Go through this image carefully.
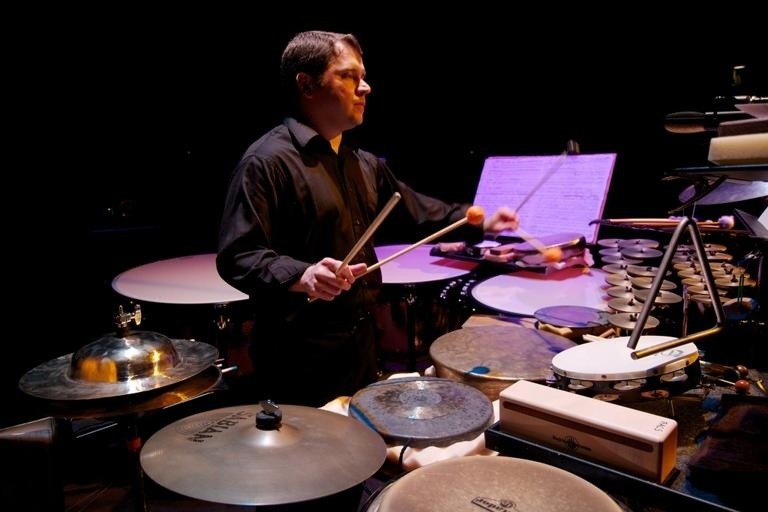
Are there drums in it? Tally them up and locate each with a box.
[369,455,630,512]
[551,334,710,416]
[350,377,495,447]
[429,324,580,403]
[368,244,497,378]
[111,252,256,381]
[470,267,621,342]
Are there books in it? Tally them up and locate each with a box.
[472,150,616,248]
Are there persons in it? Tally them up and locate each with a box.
[216,28,518,399]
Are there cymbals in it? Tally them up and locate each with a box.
[598,237,759,330]
[19,330,224,422]
[140,404,389,507]
